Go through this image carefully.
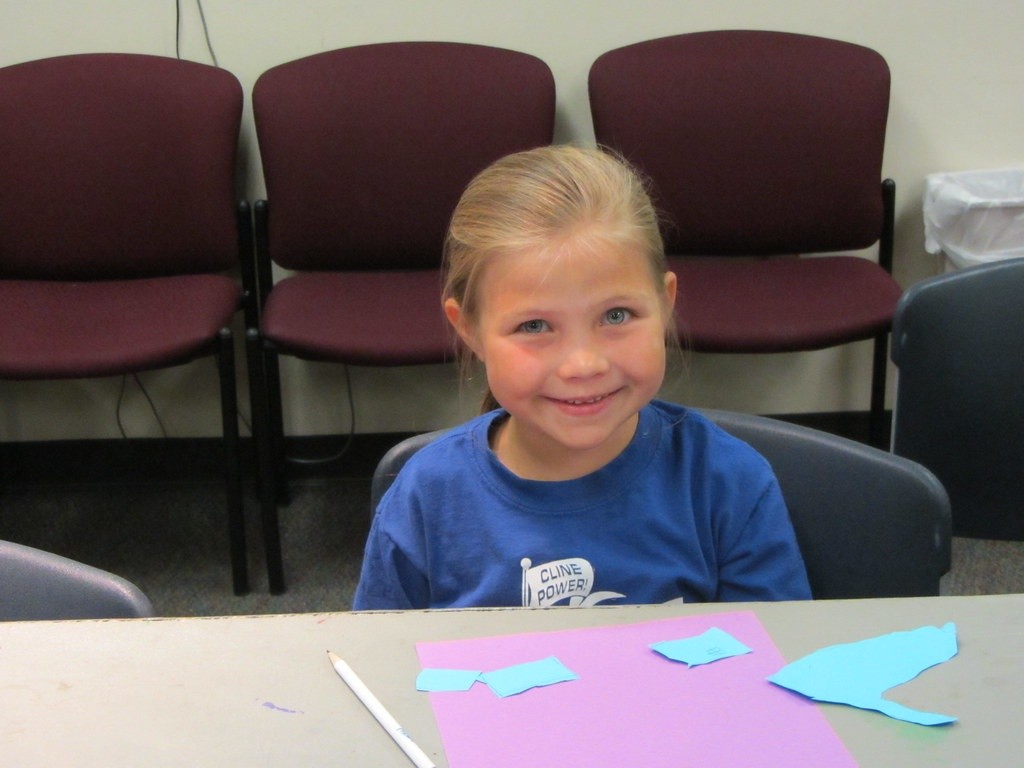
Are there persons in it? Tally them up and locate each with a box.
[353,146,813,613]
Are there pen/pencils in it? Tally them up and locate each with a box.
[326,649,436,768]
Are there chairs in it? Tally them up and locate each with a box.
[585,27,909,448]
[887,257,1024,544]
[366,404,951,600]
[0,52,252,596]
[254,41,557,600]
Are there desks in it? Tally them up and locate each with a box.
[0,594,1024,768]
